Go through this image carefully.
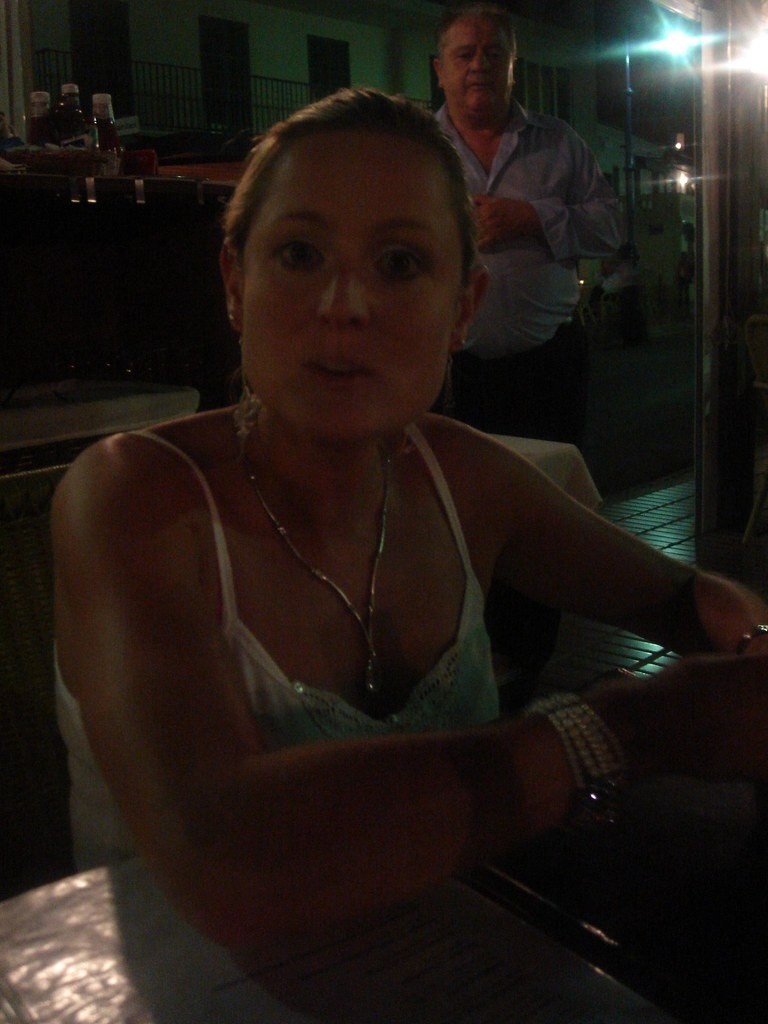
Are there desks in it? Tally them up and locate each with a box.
[485,435,602,710]
[0,772,768,1023]
[1,174,241,411]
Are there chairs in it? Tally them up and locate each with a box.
[577,282,626,329]
[741,312,768,545]
[0,464,78,898]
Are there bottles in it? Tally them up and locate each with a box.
[92,94,121,176]
[52,84,90,148]
[30,91,59,146]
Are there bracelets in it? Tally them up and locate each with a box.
[525,695,628,825]
[737,624,768,655]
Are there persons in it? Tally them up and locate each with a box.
[434,16,620,453]
[47,91,768,951]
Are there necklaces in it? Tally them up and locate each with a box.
[231,405,399,708]
